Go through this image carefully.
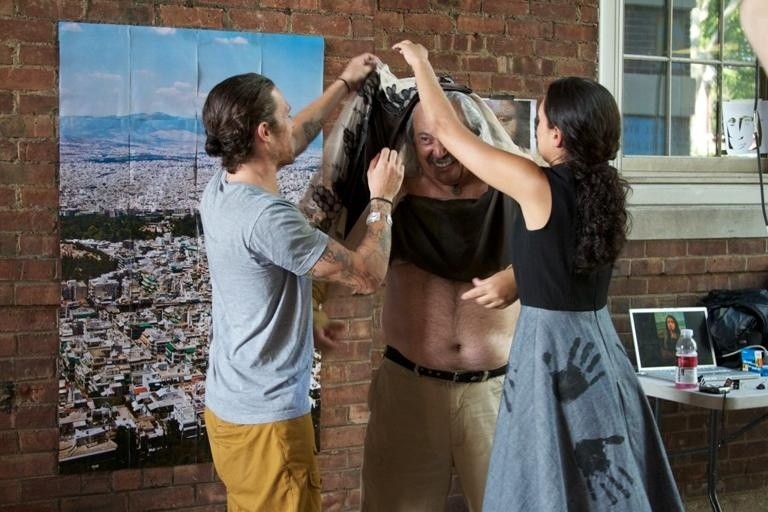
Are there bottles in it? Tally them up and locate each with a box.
[675,327,697,389]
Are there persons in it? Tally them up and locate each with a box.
[391,40,688,511]
[739,0,768,77]
[487,99,517,141]
[665,310,680,353]
[199,51,404,512]
[728,116,753,151]
[315,88,519,511]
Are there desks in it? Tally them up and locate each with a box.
[636,367,767,512]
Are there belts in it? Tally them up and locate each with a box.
[383,345,508,382]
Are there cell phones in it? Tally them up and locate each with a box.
[699,384,731,394]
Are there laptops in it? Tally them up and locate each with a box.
[628,307,761,384]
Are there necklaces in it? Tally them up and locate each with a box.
[448,182,463,196]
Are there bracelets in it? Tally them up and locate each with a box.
[338,75,352,95]
[365,211,393,227]
[367,196,395,208]
